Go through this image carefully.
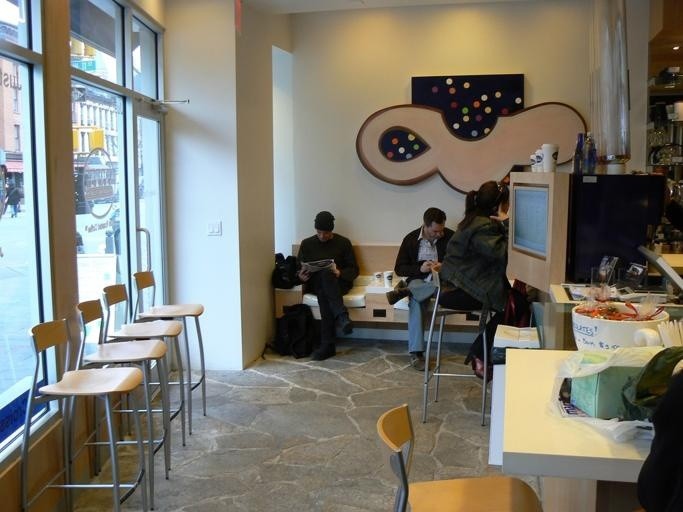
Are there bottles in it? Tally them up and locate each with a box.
[315,211,334,231]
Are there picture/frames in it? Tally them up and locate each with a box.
[485,348,683,512]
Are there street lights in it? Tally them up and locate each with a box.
[272,253,302,289]
[273,304,316,359]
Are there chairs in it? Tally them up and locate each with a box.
[312,347,336,360]
[342,319,352,335]
[409,354,431,371]
[386,288,409,305]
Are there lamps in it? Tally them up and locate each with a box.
[65,298,168,509]
[95,283,186,470]
[376,403,543,512]
[126,272,207,439]
[19,317,149,510]
[415,264,491,427]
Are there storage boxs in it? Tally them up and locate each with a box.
[273,243,515,329]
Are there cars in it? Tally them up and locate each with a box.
[528,143,559,173]
[588,264,641,301]
[650,103,682,122]
[383,270,393,289]
[374,271,382,286]
[570,302,670,351]
[668,67,680,73]
[647,217,682,253]
[646,128,671,166]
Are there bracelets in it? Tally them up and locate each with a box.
[672,156,682,163]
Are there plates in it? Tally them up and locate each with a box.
[543,283,683,348]
[646,83,683,168]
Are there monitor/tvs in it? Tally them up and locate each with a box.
[301,259,334,273]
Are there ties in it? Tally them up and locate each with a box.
[506,171,570,294]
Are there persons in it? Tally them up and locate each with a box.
[386,207,456,371]
[297,211,359,361]
[7,182,20,217]
[438,180,510,382]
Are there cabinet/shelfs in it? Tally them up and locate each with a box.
[574,132,596,176]
[665,178,682,205]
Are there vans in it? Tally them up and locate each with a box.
[464,353,490,379]
